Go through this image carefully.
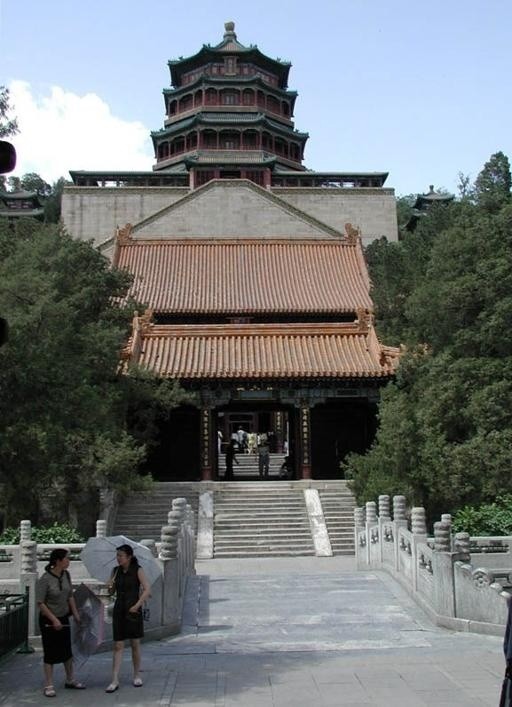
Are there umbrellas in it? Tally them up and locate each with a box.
[79,535,163,591]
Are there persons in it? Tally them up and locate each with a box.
[218,425,288,479]
[36,545,151,697]
[499,596,512,707]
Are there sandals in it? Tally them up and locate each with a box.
[44,677,144,696]
